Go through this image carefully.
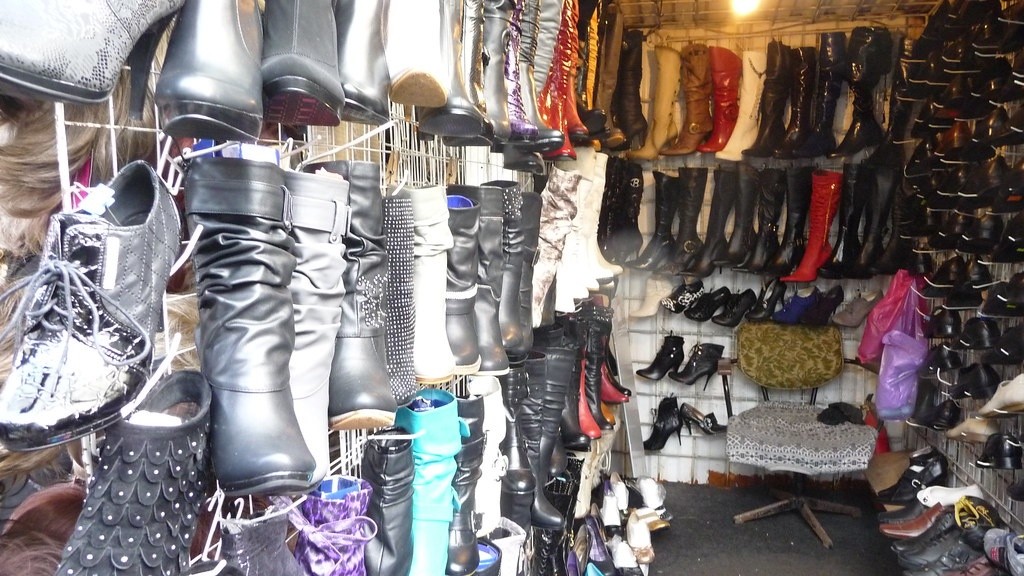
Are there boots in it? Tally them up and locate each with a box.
[156,0,624,576]
[624,27,911,283]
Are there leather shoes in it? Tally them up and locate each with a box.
[623,473,672,576]
[877,1,1024,576]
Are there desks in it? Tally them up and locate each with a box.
[726,400,880,549]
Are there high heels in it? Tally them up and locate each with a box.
[631,279,882,461]
[2,1,185,121]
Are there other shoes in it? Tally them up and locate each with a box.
[0,160,210,576]
